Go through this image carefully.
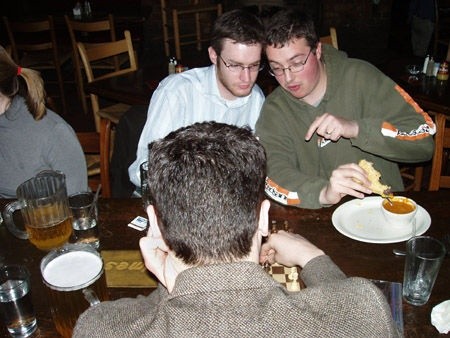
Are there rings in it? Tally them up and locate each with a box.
[325,131,332,135]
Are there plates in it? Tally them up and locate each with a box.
[332,196,431,244]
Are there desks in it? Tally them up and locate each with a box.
[396,60,450,115]
[54,6,154,55]
[87,56,207,107]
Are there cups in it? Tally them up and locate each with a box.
[68,192,102,250]
[40,243,110,338]
[402,235,446,306]
[139,161,159,213]
[0,264,39,338]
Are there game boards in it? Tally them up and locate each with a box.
[257,224,309,293]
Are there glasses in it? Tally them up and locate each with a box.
[270,44,315,77]
[216,54,264,72]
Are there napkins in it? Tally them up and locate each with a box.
[431,300,450,334]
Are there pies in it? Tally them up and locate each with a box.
[353,160,391,197]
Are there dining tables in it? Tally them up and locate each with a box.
[0,194,450,338]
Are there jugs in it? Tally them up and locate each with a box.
[2,169,73,251]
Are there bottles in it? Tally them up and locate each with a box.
[421,54,449,81]
[168,57,189,74]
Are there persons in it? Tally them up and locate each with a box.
[0,46,88,200]
[71,122,402,338]
[126,10,266,195]
[251,11,435,209]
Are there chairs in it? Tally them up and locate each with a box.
[0,0,450,197]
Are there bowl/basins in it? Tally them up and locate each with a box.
[382,196,418,225]
[408,64,419,74]
